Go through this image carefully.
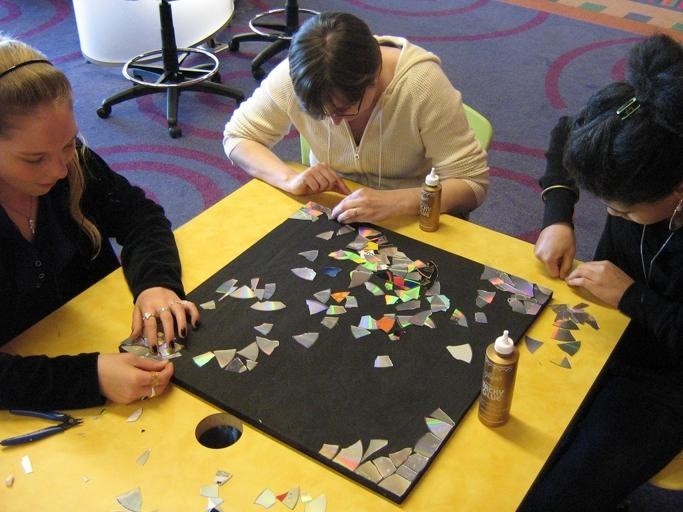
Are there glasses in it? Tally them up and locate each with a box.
[305,88,366,119]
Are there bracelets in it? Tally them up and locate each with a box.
[540,185,580,205]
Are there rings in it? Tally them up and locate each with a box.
[158,307,170,318]
[141,396,150,401]
[182,300,187,309]
[353,208,358,217]
[150,386,158,398]
[142,312,157,321]
[170,301,183,306]
[150,370,161,386]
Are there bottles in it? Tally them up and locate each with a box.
[478,329,520,429]
[419,168,443,230]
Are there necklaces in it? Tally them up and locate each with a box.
[3,187,39,235]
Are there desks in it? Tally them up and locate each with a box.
[1,162,637,512]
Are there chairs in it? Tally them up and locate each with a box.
[298,105,492,179]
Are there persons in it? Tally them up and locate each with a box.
[222,14,491,229]
[512,33,682,510]
[1,36,201,412]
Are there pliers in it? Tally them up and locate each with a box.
[0,410,82,445]
